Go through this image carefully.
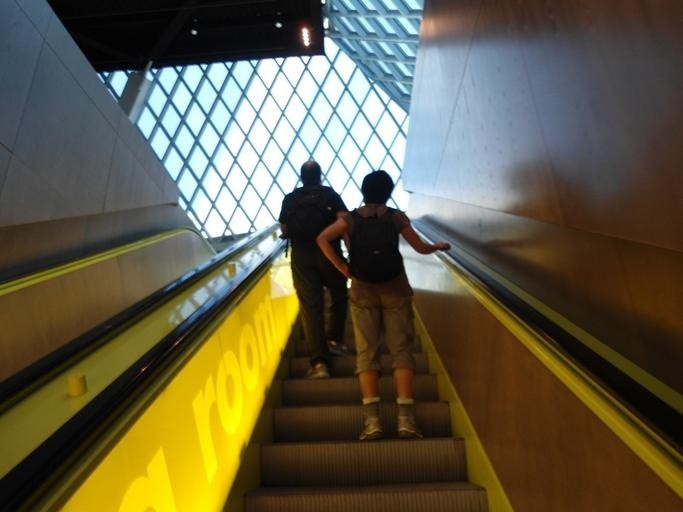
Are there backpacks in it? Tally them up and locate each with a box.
[285,189,336,246]
[346,207,404,284]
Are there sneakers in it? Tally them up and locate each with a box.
[395,414,424,439]
[310,361,330,378]
[325,339,349,355]
[357,414,385,440]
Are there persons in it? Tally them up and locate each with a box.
[277,161,348,379]
[315,169,452,440]
